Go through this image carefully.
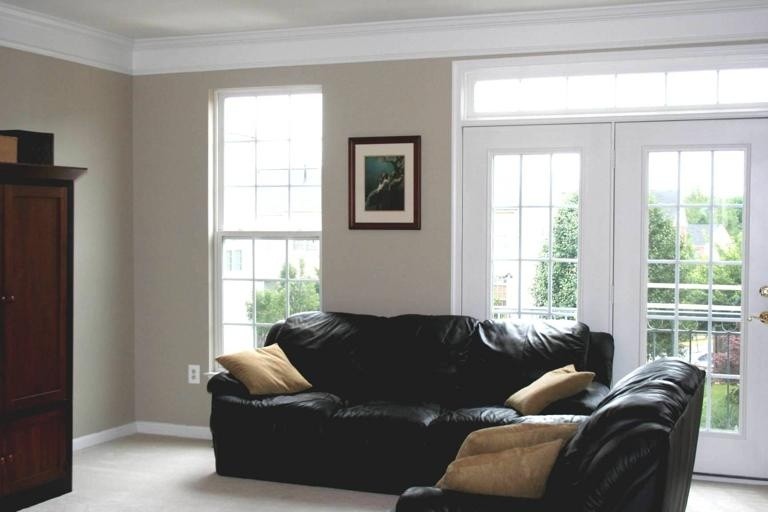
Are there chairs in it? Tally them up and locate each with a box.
[395,360,705,512]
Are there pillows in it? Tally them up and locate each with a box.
[441,440,564,499]
[455,422,578,456]
[505,365,594,415]
[215,343,312,396]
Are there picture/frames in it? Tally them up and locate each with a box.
[347,135,421,230]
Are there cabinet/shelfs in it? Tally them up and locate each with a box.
[0,183,73,512]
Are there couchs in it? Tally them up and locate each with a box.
[207,312,615,497]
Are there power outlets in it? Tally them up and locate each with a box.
[188,364,200,384]
